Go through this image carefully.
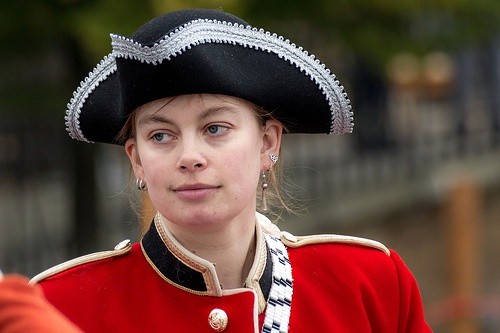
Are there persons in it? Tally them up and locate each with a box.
[0,271,86,333]
[29,8,434,333]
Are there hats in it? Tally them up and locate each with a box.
[63,8,354,145]
